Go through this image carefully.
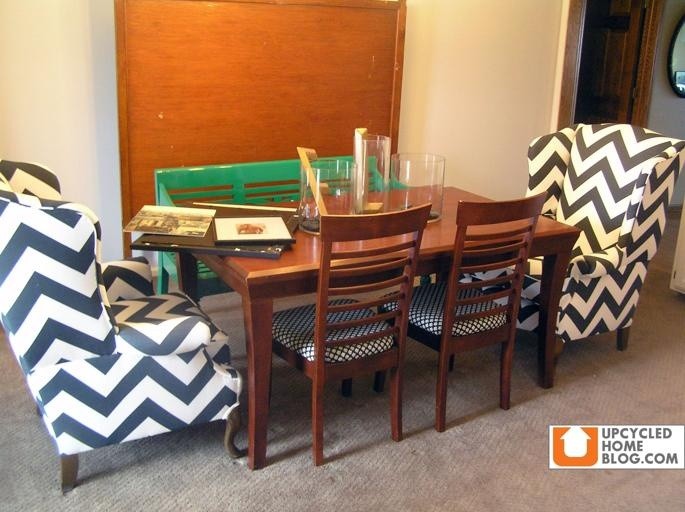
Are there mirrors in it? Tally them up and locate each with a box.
[667,15,685,98]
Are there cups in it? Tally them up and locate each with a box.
[351,133,392,216]
[394,150,448,224]
[297,154,361,238]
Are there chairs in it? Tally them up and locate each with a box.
[270,203,433,464]
[374,192,549,432]
[0,158,249,492]
[453,120,685,356]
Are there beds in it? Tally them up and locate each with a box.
[180,185,580,470]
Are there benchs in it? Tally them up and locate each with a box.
[154,154,408,309]
